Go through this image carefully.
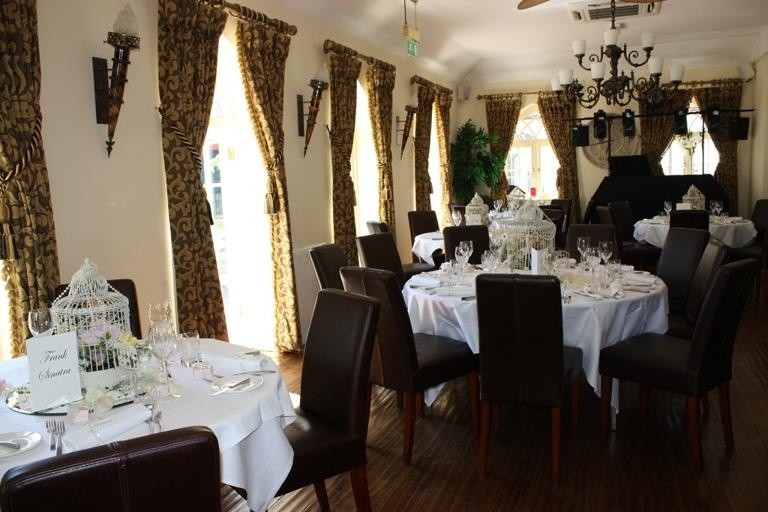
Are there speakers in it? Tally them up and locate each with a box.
[672,108,688,135]
[594,110,607,140]
[730,117,749,140]
[571,125,588,146]
[622,109,636,137]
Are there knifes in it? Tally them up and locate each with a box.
[212,375,251,396]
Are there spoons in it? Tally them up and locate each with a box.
[155,411,161,433]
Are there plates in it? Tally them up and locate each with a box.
[0,431,42,458]
[63,419,153,451]
[211,372,265,395]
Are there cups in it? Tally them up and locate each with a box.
[150,321,182,400]
[715,201,723,217]
[709,200,716,214]
[147,299,173,325]
[480,253,491,266]
[178,331,202,368]
[450,209,463,227]
[494,199,500,211]
[454,246,470,267]
[598,240,614,266]
[576,237,591,259]
[663,201,673,219]
[27,306,56,339]
[459,240,474,260]
[584,245,602,272]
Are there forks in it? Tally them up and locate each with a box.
[45,417,59,452]
[53,421,66,457]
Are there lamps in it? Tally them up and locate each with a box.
[568,117,590,147]
[727,115,750,140]
[622,108,636,142]
[396,94,418,160]
[593,110,607,145]
[298,59,328,160]
[552,1,685,109]
[90,0,142,159]
[673,107,686,135]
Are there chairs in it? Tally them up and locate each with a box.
[226,287,381,512]
[55,277,144,342]
[453,206,466,225]
[753,200,768,236]
[599,257,762,475]
[539,205,564,236]
[309,243,347,290]
[1,425,222,512]
[338,265,480,459]
[550,198,572,228]
[608,200,634,241]
[408,210,441,263]
[444,225,489,268]
[356,233,405,293]
[472,273,586,482]
[597,205,617,225]
[565,222,618,265]
[657,228,711,311]
[365,222,435,285]
[638,241,730,420]
[668,209,709,230]
